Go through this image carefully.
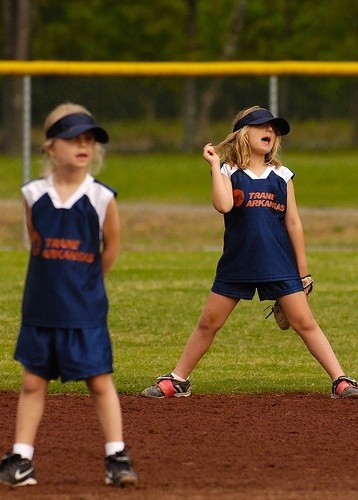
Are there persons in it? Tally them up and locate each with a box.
[0,101,138,489]
[141,105,358,399]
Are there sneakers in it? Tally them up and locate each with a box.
[0,451,37,487]
[141,374,192,398]
[104,448,138,488]
[331,376,358,399]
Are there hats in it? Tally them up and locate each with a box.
[46,112,109,144]
[234,110,290,136]
